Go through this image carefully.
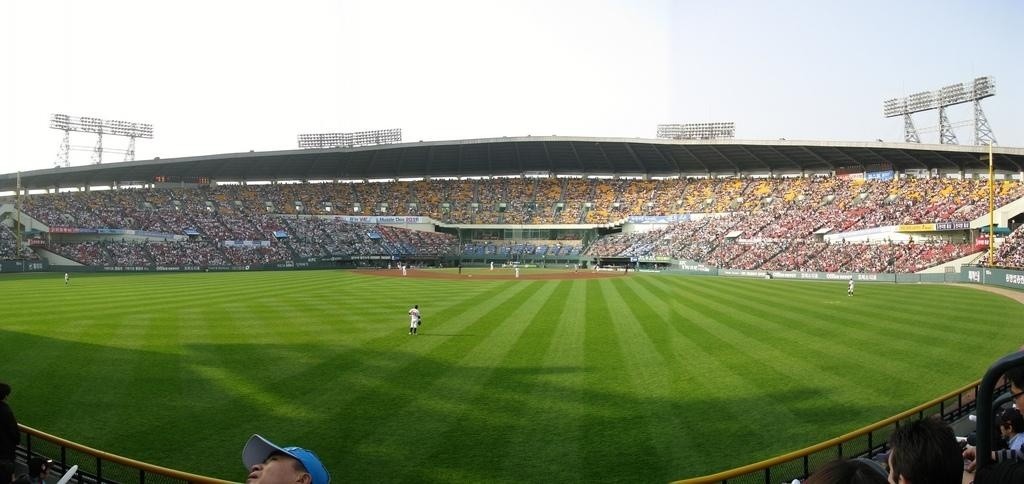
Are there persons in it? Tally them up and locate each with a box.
[1,183,460,266]
[800,460,890,484]
[589,173,1023,274]
[0,382,21,483]
[406,303,420,335]
[847,278,855,296]
[63,270,70,287]
[962,365,1024,484]
[884,414,965,484]
[999,405,1024,449]
[387,262,630,277]
[386,174,617,254]
[0,460,18,483]
[24,454,55,484]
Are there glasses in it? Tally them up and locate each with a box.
[1009,391,1024,404]
